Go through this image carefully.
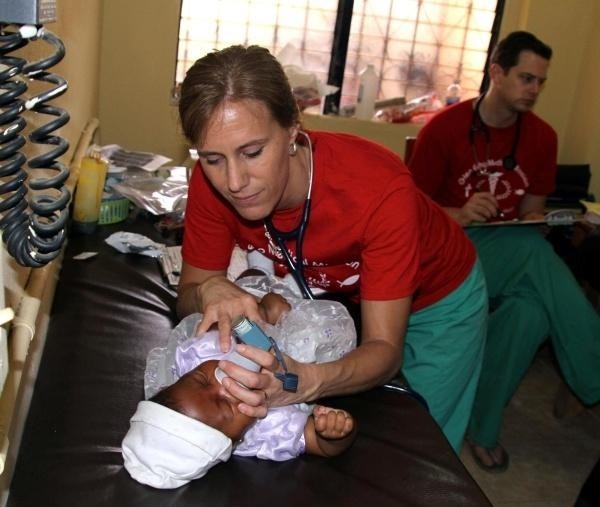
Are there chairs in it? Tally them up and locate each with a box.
[406,136,600,372]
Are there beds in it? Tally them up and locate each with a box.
[0,117,492,505]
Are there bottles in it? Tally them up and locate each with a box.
[355,64,378,120]
[446,83,461,108]
[71,148,109,232]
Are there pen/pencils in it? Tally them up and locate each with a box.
[475,187,505,218]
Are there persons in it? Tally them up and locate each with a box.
[122,275,357,489]
[408,31,599,473]
[176,44,489,459]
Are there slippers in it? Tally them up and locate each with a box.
[465,431,509,473]
